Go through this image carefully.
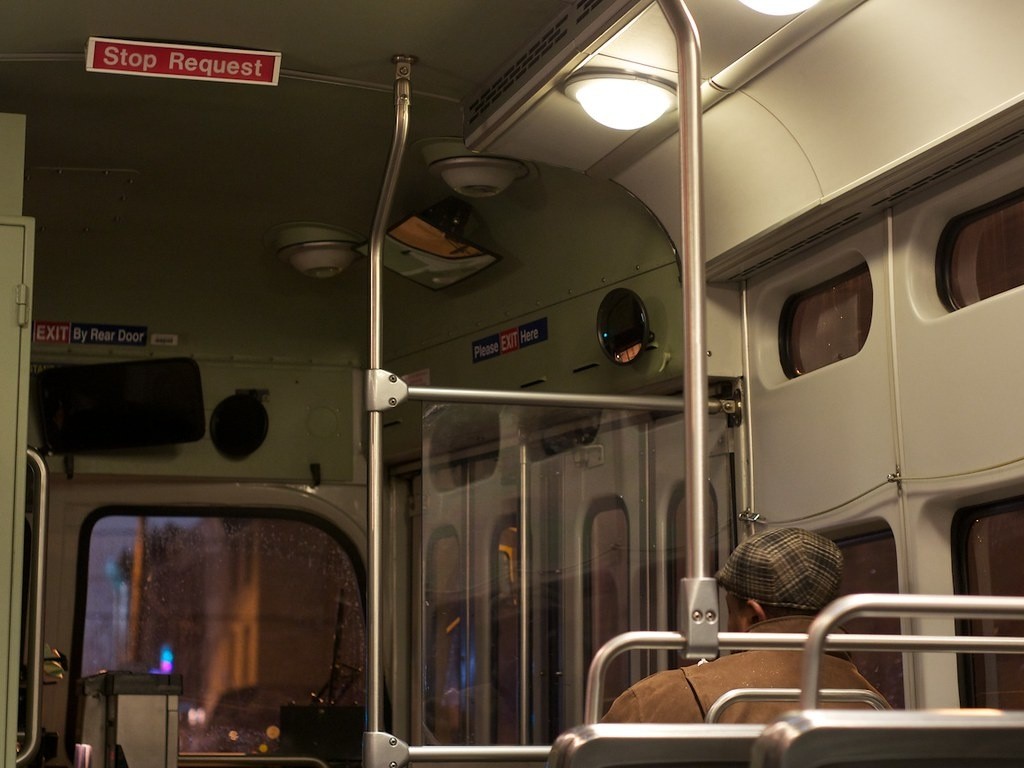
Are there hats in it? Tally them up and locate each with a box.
[712,527,845,612]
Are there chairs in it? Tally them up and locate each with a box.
[547,593,1024,768]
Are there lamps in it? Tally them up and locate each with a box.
[409,136,539,199]
[262,221,368,279]
[564,71,677,134]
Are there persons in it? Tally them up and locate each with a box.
[601,526,892,725]
